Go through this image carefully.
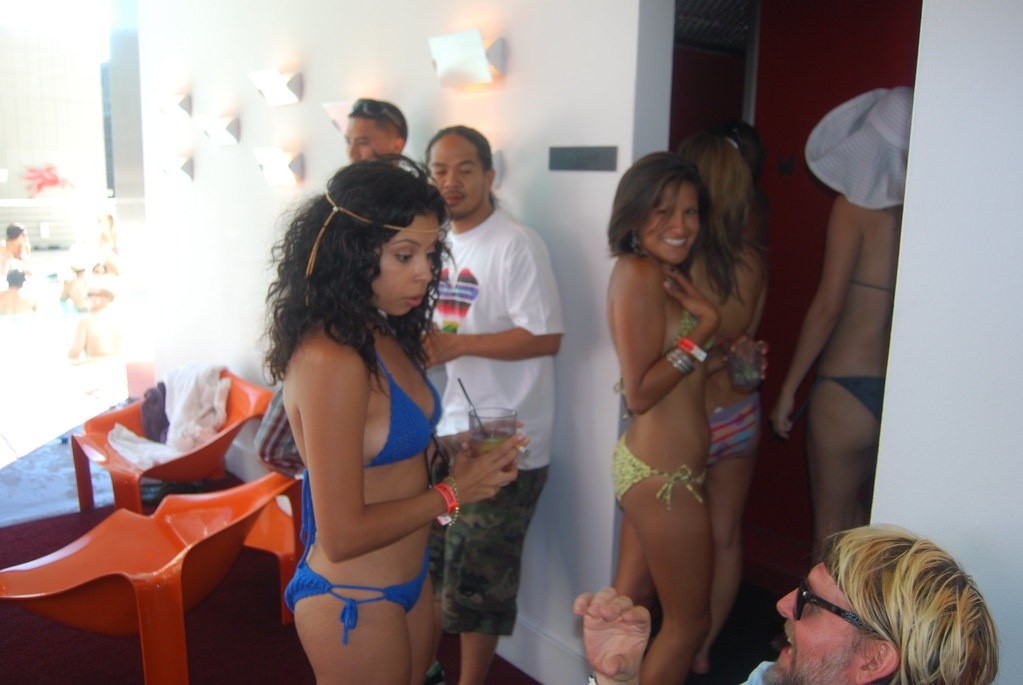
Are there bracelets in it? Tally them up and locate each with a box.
[678,337,708,363]
[665,347,696,376]
[434,476,462,528]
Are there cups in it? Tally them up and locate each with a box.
[469,408,517,487]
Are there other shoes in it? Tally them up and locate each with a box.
[423,660,446,685]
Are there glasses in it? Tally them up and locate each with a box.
[706,121,746,156]
[431,433,449,484]
[349,99,406,135]
[793,577,881,632]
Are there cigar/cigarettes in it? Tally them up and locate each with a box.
[514,443,528,454]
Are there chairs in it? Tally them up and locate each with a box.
[0,469,308,684]
[72,367,274,516]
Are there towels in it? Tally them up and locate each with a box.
[105,364,235,471]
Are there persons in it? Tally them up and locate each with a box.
[1,213,122,365]
[605,152,768,685]
[672,86,916,675]
[572,522,1001,685]
[254,99,566,685]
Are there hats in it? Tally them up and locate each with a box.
[805,86,914,211]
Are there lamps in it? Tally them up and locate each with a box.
[430,28,506,84]
